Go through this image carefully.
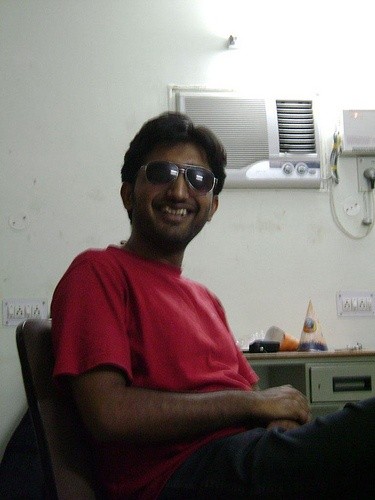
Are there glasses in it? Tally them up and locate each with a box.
[137,159,220,194]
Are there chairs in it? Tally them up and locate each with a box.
[15,318,104,500]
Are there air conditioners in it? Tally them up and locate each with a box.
[176,92,322,189]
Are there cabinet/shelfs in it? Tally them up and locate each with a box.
[243,350,375,417]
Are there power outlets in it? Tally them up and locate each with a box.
[336,292,375,316]
[2,298,48,326]
[356,157,375,192]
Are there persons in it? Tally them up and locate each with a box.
[50,111,375,500]
